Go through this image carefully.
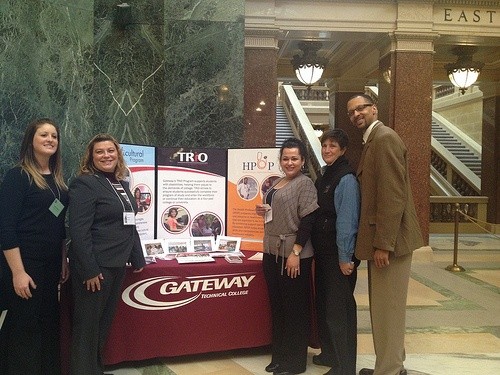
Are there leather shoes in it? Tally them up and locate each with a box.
[266,362,280,372]
[273,366,306,375]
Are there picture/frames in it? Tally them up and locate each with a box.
[164,239,191,254]
[216,235,241,253]
[191,236,216,253]
[140,238,165,257]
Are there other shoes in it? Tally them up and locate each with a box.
[323,366,337,375]
[313,355,337,366]
[359,368,406,375]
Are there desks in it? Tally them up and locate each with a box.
[58,251,320,371]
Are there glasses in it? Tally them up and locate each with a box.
[346,104,372,117]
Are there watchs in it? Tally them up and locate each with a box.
[292,250,300,256]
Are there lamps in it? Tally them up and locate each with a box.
[444,45,484,95]
[290,41,329,86]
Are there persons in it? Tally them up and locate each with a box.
[310,129,361,375]
[0,118,70,375]
[68,133,145,375]
[238,177,250,198]
[146,241,235,255]
[346,93,425,375]
[256,138,319,375]
[164,208,186,232]
[134,187,152,211]
[192,214,221,238]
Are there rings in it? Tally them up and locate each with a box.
[349,270,351,273]
